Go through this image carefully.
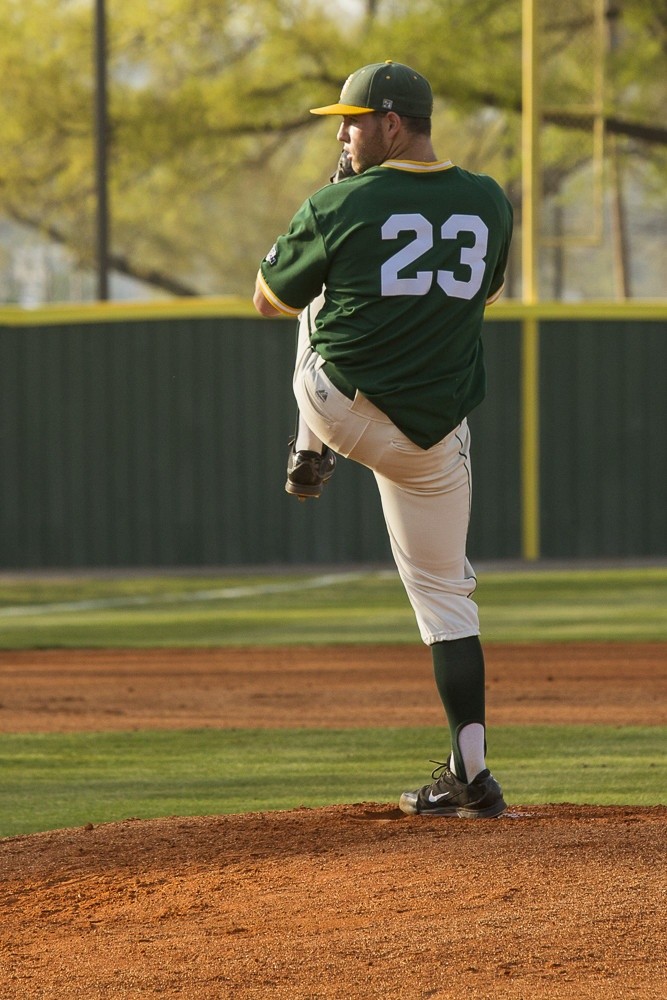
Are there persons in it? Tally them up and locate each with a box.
[252,58,512,819]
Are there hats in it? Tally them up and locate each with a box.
[310,59,432,117]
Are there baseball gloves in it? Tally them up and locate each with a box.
[328,147,360,183]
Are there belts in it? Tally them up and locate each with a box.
[321,362,357,401]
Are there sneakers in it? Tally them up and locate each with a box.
[284,439,336,500]
[399,753,507,817]
